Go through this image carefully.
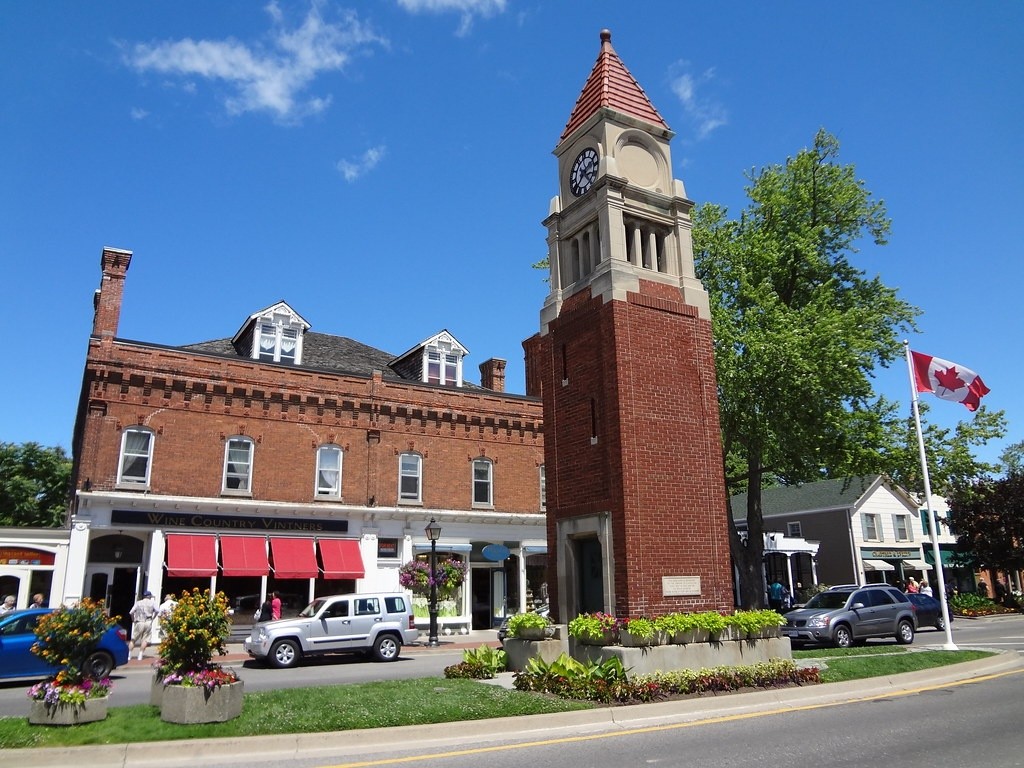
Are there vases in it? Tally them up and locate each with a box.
[157,684,255,722]
[29,699,109,723]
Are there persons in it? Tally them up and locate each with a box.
[978,577,990,598]
[158,595,178,643]
[29,594,44,627]
[895,577,933,598]
[253,591,281,621]
[766,578,797,614]
[0,595,16,617]
[995,579,1005,603]
[128,591,159,661]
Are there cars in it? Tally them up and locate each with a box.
[0,608,129,683]
[903,594,954,631]
[497,605,549,646]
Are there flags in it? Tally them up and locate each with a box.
[909,349,991,413]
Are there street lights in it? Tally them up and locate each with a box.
[424,516,443,647]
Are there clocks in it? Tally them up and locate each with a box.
[566,145,600,198]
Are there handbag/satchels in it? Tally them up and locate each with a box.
[254,609,262,621]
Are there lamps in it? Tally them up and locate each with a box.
[115,530,126,561]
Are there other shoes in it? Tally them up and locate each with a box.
[128,657,132,660]
[138,657,142,660]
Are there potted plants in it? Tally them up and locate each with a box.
[621,609,789,649]
[569,613,616,646]
[502,614,557,639]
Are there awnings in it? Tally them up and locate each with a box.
[167,534,217,577]
[220,535,269,576]
[412,542,472,551]
[319,539,365,578]
[521,538,547,553]
[271,538,318,579]
[902,560,933,570]
[926,550,973,568]
[862,560,895,571]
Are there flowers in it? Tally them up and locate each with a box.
[397,556,470,592]
[148,587,245,696]
[598,610,628,640]
[26,595,119,710]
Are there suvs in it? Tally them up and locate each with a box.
[780,583,918,649]
[243,592,418,668]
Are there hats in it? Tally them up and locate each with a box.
[143,591,151,596]
